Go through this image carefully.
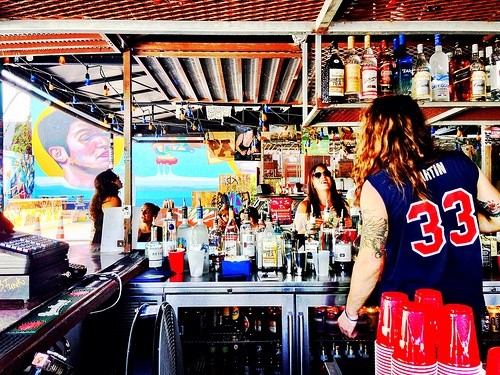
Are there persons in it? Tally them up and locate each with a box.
[337,95,500,347]
[294,163,353,234]
[235,128,257,160]
[216,206,261,235]
[137,203,162,242]
[90,168,124,243]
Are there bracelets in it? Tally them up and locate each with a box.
[345,307,359,321]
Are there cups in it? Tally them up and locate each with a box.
[187,250,204,278]
[373,288,500,375]
[168,248,185,274]
[496,255,500,269]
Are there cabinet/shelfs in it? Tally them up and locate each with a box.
[165,294,379,375]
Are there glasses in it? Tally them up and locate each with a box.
[312,170,331,178]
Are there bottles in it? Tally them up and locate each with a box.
[323,34,412,104]
[181,307,371,375]
[429,34,450,102]
[191,206,209,274]
[241,208,255,264]
[176,205,192,272]
[209,218,223,272]
[145,224,163,269]
[162,207,176,260]
[480,231,500,274]
[224,205,240,258]
[256,206,362,277]
[412,44,431,102]
[447,40,500,102]
